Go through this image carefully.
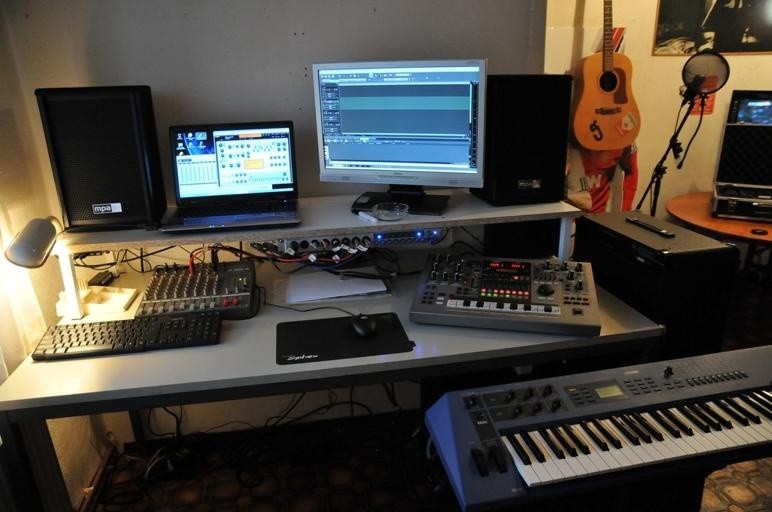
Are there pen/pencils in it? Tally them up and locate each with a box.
[356,210,379,222]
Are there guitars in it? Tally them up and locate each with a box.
[571,0,641,152]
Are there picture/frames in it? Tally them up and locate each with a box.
[651,0,772,56]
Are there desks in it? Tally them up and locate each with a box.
[1,187,668,512]
[666,191,771,291]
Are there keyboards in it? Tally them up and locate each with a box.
[32,312,223,361]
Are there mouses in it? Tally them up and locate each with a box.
[350,313,373,338]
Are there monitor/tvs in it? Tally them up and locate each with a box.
[312,56,487,215]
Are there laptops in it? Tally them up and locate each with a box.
[158,120,300,234]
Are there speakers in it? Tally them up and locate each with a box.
[34,85,167,231]
[470,73,571,203]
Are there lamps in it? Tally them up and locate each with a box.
[4,215,91,317]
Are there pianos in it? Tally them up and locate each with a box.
[425,346,772,511]
